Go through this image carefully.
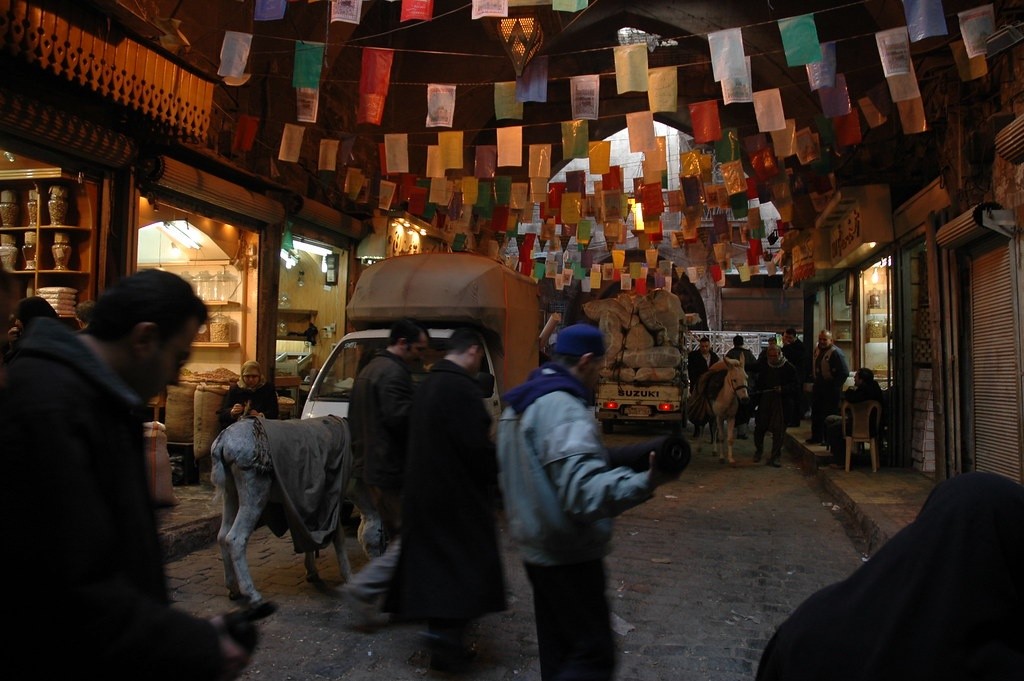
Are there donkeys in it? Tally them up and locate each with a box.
[696,351,748,468]
[210,414,359,608]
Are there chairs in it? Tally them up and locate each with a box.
[842,400,881,473]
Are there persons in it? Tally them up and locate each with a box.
[756,473,1024,681]
[0,268,249,681]
[497,326,690,681]
[337,318,431,632]
[687,328,885,468]
[8,296,61,345]
[220,362,279,429]
[382,320,504,675]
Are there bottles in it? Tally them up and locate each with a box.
[0,187,72,271]
[181,270,238,343]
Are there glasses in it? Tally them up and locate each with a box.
[243,374,259,379]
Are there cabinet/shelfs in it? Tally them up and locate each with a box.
[833,319,853,341]
[275,309,318,421]
[0,167,99,330]
[866,310,893,378]
[137,260,240,347]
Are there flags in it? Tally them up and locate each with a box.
[156,0,996,295]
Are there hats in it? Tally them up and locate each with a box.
[556,324,606,357]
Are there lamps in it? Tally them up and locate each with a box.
[162,221,202,250]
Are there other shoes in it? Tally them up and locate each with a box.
[829,461,845,469]
[339,582,387,623]
[806,436,823,444]
[753,450,782,467]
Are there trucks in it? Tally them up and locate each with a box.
[593,318,689,438]
[298,251,539,522]
